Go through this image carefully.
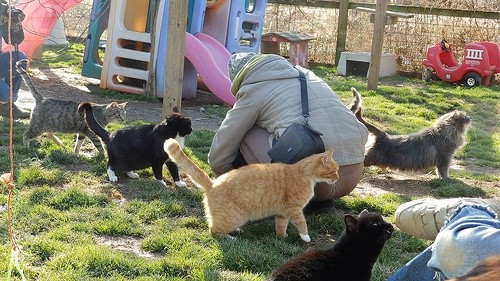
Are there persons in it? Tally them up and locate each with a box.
[208,52,369,210]
[0,13,28,104]
[386,197,500,281]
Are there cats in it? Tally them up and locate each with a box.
[345,85,473,181]
[75,101,193,187]
[266,208,394,281]
[14,58,129,153]
[161,135,339,243]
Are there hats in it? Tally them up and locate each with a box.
[226,52,256,83]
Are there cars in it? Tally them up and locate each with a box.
[422,38,500,89]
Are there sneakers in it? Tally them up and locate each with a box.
[394,198,500,241]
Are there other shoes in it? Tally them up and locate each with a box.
[0,102,30,118]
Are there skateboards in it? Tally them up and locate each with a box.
[0,0,83,74]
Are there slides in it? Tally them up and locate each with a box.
[183,31,238,106]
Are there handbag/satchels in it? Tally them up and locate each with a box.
[266,122,326,164]
